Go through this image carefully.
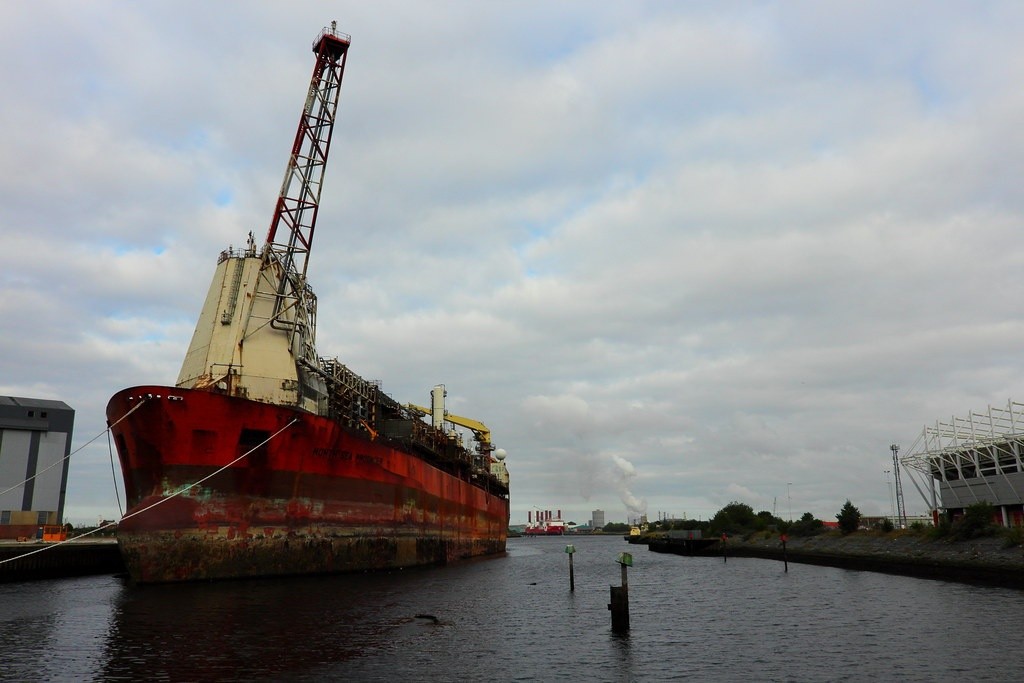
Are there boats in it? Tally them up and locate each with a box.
[525,509,569,535]
[106,22,511,580]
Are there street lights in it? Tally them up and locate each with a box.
[788,482,793,522]
